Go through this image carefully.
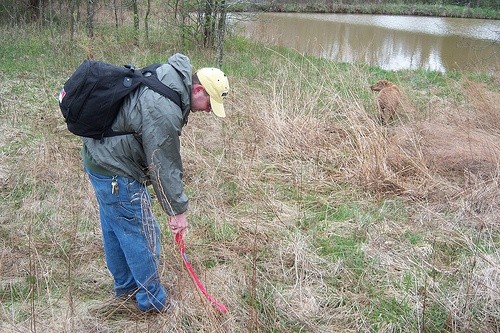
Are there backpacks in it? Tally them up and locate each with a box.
[58,59,183,141]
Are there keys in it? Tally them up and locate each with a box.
[111,175,119,195]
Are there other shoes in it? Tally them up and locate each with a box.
[160,299,174,314]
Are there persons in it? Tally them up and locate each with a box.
[82,53,230,314]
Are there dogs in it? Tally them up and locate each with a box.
[370,79,402,125]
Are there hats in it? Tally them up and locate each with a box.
[195,67,229,118]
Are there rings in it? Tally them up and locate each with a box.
[173,226,175,230]
